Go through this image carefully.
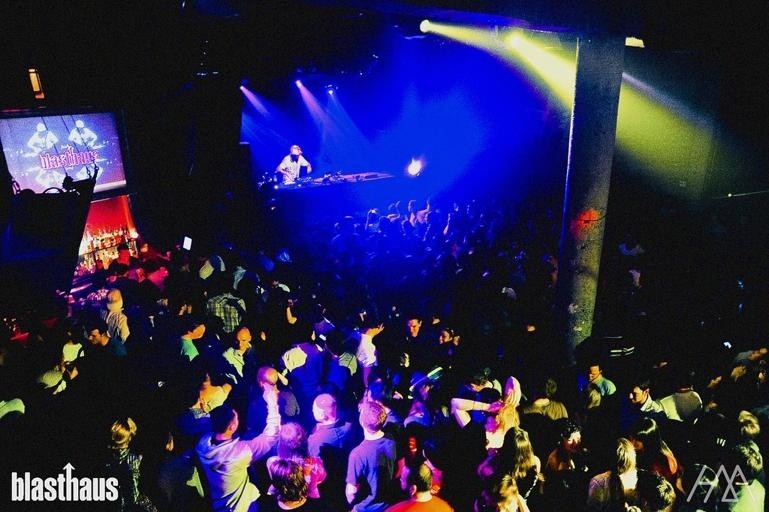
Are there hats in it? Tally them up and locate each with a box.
[315,392,337,422]
[106,288,124,311]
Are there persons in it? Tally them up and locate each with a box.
[1,190,767,512]
[24,122,58,154]
[66,120,99,150]
[275,144,312,185]
